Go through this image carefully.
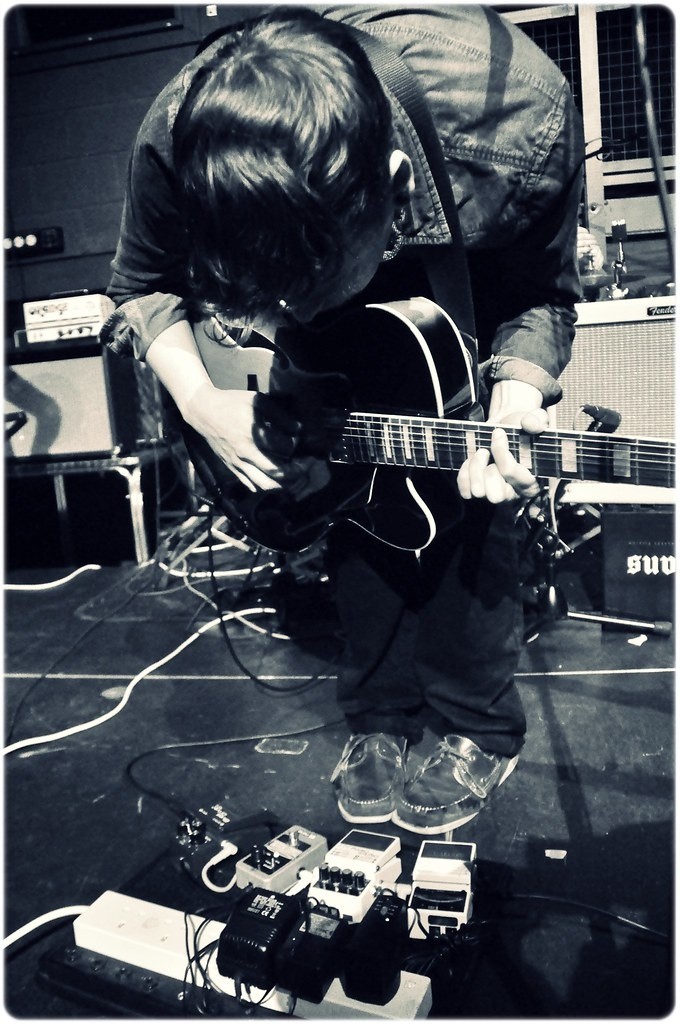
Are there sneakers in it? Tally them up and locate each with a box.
[329,732,408,824]
[391,734,520,835]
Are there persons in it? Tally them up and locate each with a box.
[97,4,584,833]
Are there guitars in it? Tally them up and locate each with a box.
[167,292,676,557]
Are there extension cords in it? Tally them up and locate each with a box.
[72,889,433,1021]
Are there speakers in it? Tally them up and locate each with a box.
[4,336,140,459]
[545,294,676,506]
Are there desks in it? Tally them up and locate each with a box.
[5,448,171,577]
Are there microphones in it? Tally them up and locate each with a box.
[580,404,621,427]
[4,412,26,423]
